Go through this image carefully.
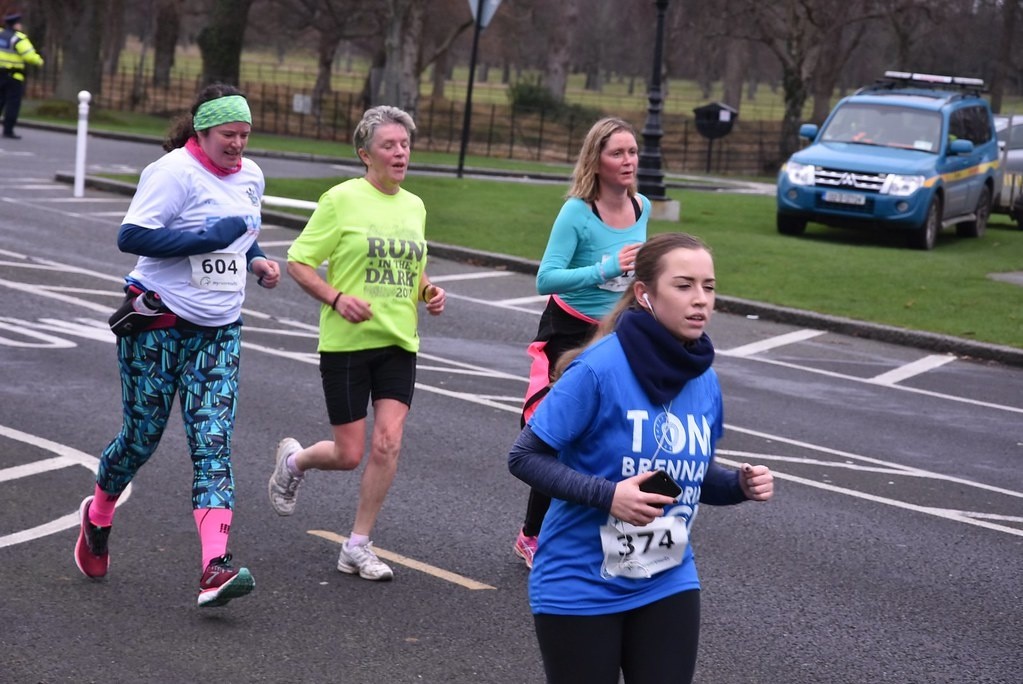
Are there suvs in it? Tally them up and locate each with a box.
[775,68,1002,252]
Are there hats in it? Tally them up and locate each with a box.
[5,12,22,25]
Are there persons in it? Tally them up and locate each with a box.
[511,116,651,569]
[267,106,447,580]
[73,83,280,606]
[0,12,44,138]
[506,233,775,684]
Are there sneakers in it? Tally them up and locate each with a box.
[337,539,393,580]
[266,437,307,516]
[73,495,112,580]
[198,552,255,608]
[514,526,540,570]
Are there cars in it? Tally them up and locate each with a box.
[993,115,1023,228]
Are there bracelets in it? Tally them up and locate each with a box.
[422,284,430,303]
[332,292,342,310]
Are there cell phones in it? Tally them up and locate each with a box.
[639,470,682,509]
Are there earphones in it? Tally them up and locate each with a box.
[643,293,652,310]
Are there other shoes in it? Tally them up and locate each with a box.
[3,130,21,138]
